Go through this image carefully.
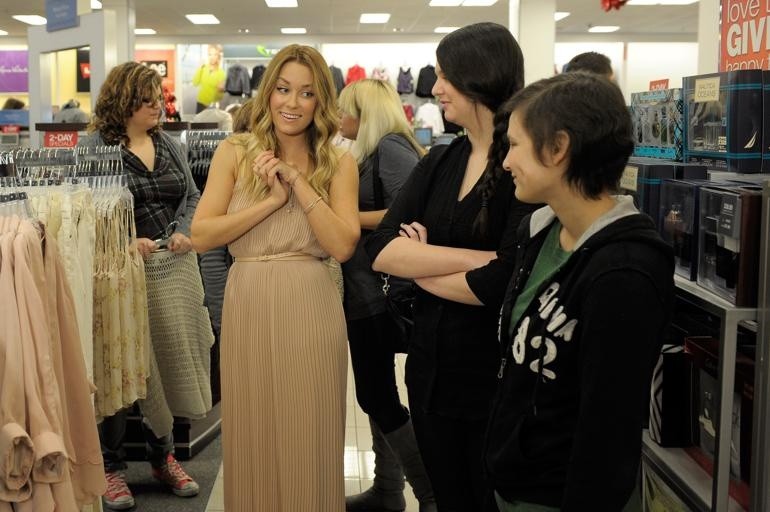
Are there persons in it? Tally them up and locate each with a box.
[192,104,242,131]
[484,72,677,512]
[191,45,360,512]
[365,23,547,512]
[75,62,200,510]
[191,43,227,114]
[3,98,25,111]
[563,52,616,84]
[337,79,439,512]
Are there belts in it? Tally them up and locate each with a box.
[234,252,321,262]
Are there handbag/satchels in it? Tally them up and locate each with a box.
[342,146,416,356]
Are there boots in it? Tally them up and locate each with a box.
[381,414,437,512]
[346,405,409,511]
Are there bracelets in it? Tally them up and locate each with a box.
[303,196,323,214]
[291,171,302,187]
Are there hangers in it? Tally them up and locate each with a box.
[0,141,134,244]
[176,129,229,175]
[35,121,219,131]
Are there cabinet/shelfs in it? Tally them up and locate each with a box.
[625,153,770,512]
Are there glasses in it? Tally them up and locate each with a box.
[134,94,165,107]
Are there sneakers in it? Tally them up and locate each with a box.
[153,455,200,496]
[101,470,135,509]
